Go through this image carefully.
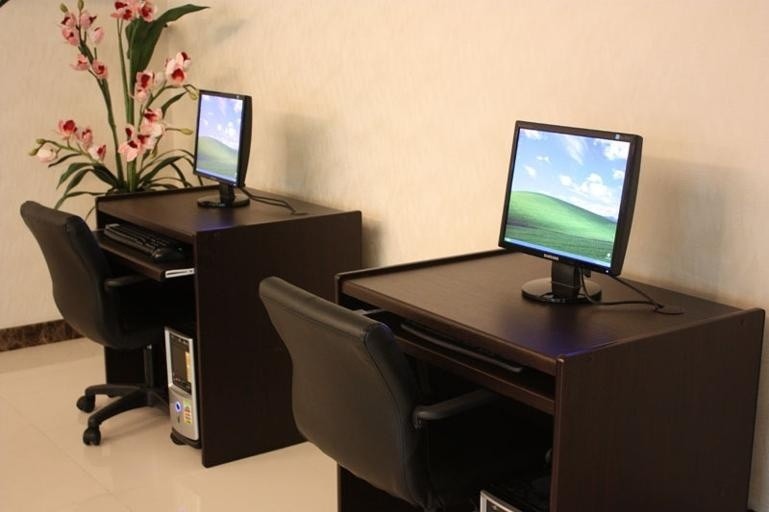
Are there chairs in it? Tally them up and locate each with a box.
[20,201,181,446]
[260,276,531,512]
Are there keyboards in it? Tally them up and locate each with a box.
[103,222,180,260]
[400,320,524,373]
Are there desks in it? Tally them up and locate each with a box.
[335,249,765,512]
[96,183,363,468]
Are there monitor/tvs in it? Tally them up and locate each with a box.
[497,119,644,308]
[192,89,253,209]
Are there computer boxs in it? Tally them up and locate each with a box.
[164,326,199,441]
[480,490,555,512]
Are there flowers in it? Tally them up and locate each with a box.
[31,0,211,221]
